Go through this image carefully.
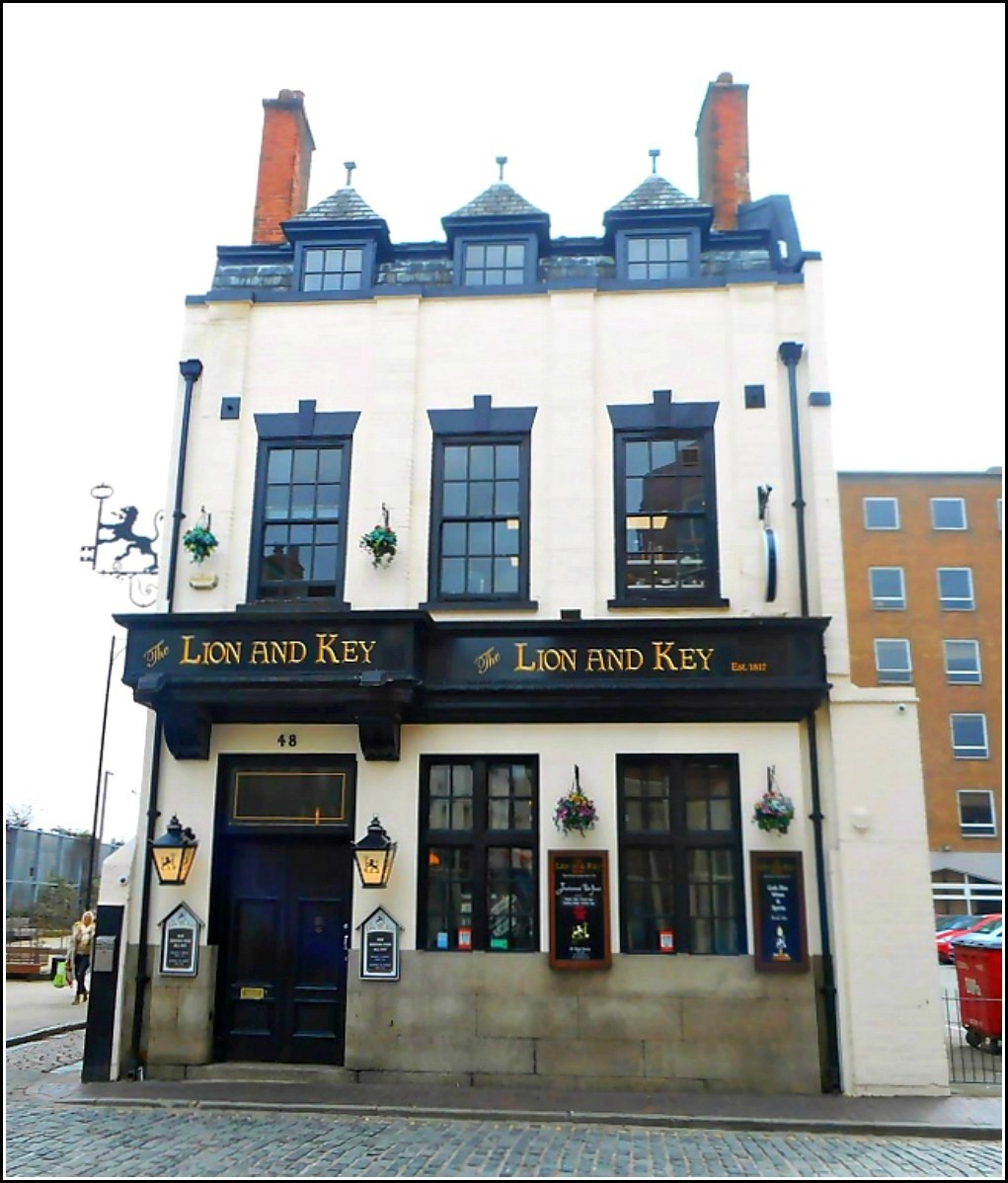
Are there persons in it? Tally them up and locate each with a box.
[66,911,95,1005]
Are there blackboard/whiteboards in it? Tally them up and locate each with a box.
[751,851,811,972]
[547,849,612,970]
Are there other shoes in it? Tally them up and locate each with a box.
[84,990,89,1002]
[72,996,80,1006]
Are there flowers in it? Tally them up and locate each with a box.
[359,524,398,568]
[182,525,218,566]
[553,790,598,836]
[749,793,796,835]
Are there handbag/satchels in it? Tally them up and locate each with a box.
[53,960,73,988]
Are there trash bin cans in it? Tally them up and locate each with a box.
[952,940,1002,1056]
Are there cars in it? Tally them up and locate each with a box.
[936,913,1002,965]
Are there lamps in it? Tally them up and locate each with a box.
[851,807,871,828]
[350,816,397,889]
[149,814,199,886]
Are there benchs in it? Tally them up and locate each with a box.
[6,947,52,982]
